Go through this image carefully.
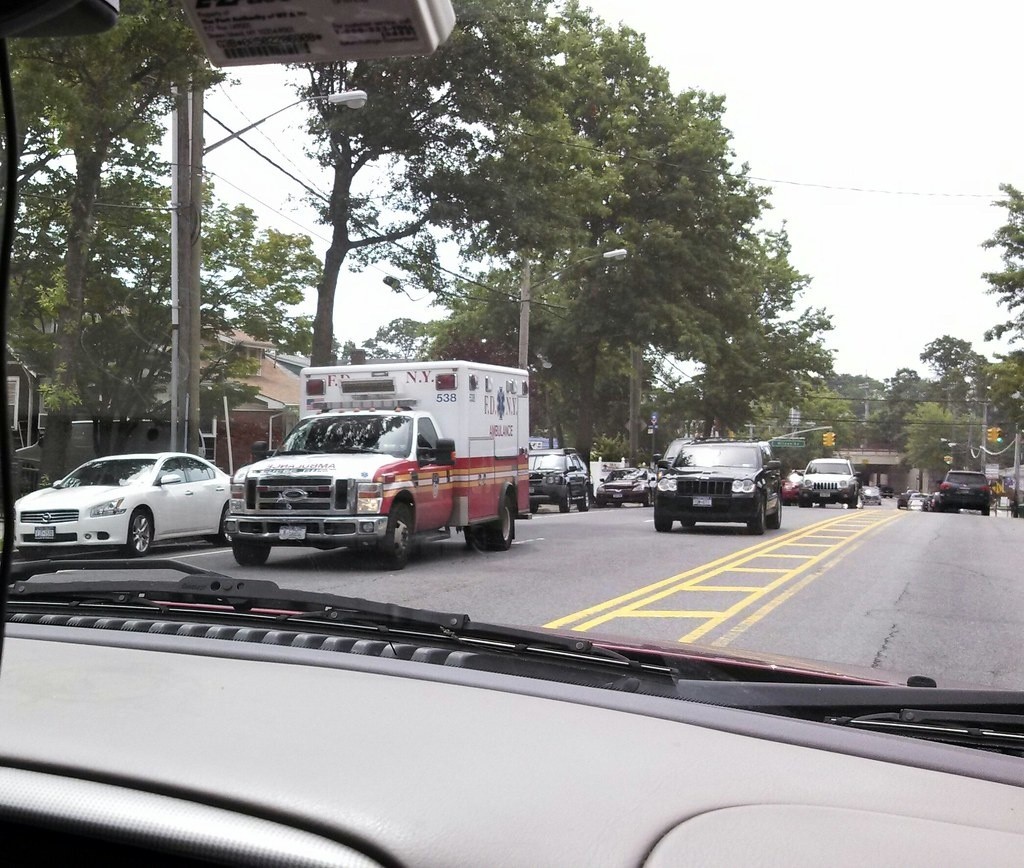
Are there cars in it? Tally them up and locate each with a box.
[595,466,659,507]
[12,451,236,561]
[858,483,895,506]
[781,469,805,506]
[896,489,960,513]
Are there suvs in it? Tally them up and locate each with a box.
[940,469,996,516]
[527,448,590,512]
[652,436,695,481]
[653,437,785,534]
[797,458,863,509]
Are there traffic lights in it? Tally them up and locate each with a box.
[987,428,994,444]
[828,432,836,447]
[993,427,1003,444]
[823,432,829,447]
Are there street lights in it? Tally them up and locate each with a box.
[517,248,629,367]
[947,442,987,474]
[187,88,369,454]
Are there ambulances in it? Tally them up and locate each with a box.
[221,358,532,567]
